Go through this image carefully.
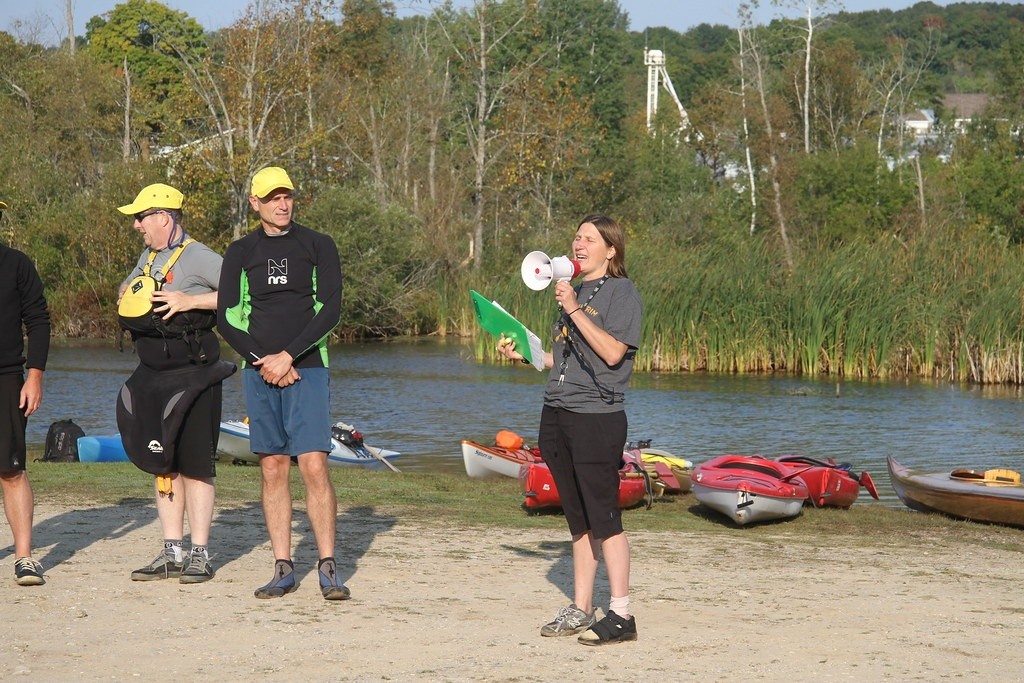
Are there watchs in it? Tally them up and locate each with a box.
[521,359,529,364]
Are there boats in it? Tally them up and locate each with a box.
[459,437,692,511]
[772,454,860,507]
[217,420,402,466]
[886,452,1023,527]
[692,454,809,527]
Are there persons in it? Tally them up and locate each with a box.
[0,201,51,586]
[497,214,638,645]
[116,183,224,583]
[215,167,349,599]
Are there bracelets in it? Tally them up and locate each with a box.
[569,308,581,316]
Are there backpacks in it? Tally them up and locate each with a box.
[117,238,196,333]
[44,419,85,464]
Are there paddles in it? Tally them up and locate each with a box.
[619,472,659,478]
[363,443,401,472]
[858,472,880,502]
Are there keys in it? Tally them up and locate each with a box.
[557,369,565,386]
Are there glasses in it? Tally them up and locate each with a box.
[134,211,171,223]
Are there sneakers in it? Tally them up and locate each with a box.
[578,610,637,646]
[130,542,186,581]
[254,559,295,599]
[541,603,596,637]
[14,556,45,585]
[318,557,349,599]
[180,545,215,585]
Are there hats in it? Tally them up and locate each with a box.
[0,201,8,209]
[250,167,294,198]
[117,184,184,215]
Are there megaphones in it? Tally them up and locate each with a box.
[521,251,581,310]
